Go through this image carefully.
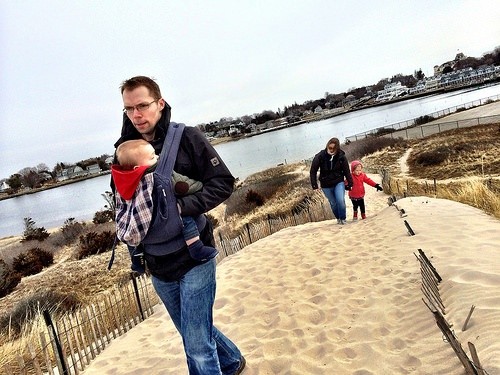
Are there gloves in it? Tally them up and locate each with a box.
[312,184,318,189]
[374,184,384,192]
[345,181,353,190]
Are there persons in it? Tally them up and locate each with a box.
[309,137,354,225]
[345,159,383,221]
[109,75,247,375]
[109,138,222,277]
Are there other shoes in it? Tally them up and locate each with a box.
[222,356,246,375]
[341,220,347,225]
[129,252,146,274]
[337,219,341,224]
[188,240,220,262]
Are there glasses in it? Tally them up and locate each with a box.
[329,146,335,150]
[123,101,156,114]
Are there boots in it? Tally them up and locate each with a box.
[353,211,357,218]
[361,213,367,219]
